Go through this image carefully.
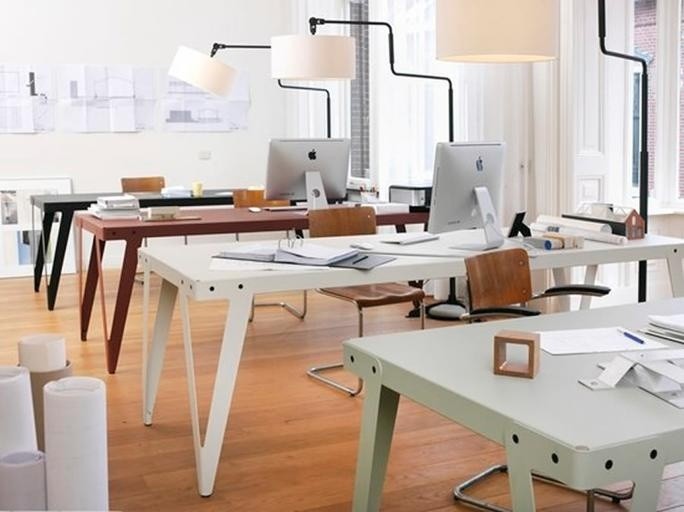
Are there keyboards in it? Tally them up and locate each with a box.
[379,232,440,247]
[264,205,308,211]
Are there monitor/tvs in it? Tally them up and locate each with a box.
[263,136,352,218]
[425,141,505,251]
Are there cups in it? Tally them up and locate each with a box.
[193,182,204,197]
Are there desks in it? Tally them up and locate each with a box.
[72,207,429,375]
[137,226,684,497]
[30,189,347,310]
[342,297,684,512]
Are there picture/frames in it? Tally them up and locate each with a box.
[508,211,531,238]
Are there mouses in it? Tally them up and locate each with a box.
[248,207,261,213]
[350,241,375,250]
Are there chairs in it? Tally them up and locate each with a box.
[121,177,188,285]
[233,190,307,322]
[453,247,635,512]
[306,206,425,396]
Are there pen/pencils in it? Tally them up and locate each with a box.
[617,328,644,346]
[352,256,368,264]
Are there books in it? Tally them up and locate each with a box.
[213,241,359,266]
[637,313,684,345]
[88,197,141,221]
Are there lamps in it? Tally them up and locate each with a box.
[271,18,468,322]
[435,0,649,303]
[168,42,331,138]
